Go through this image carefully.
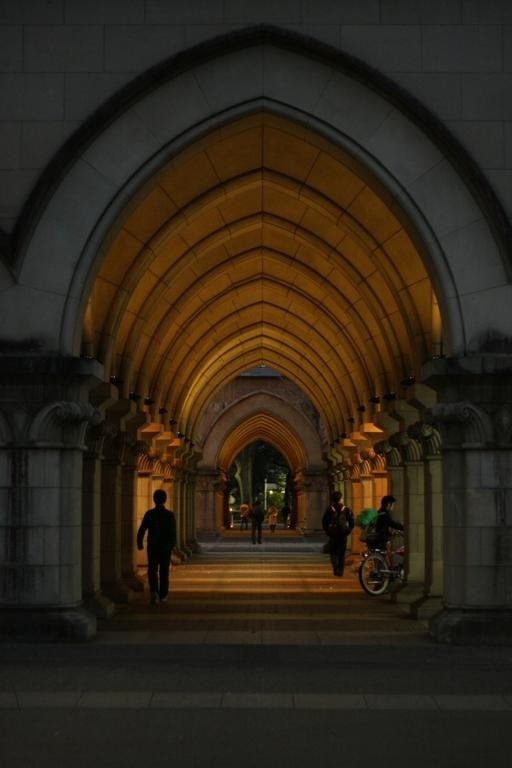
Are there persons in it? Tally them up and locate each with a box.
[365,496,404,591]
[322,492,354,576]
[137,489,176,604]
[239,501,291,544]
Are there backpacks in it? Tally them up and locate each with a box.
[356,508,386,541]
[329,504,348,535]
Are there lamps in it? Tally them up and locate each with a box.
[106,372,197,451]
[326,372,419,450]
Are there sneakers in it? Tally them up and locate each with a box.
[150,592,166,602]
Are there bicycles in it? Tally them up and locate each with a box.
[354,529,405,598]
